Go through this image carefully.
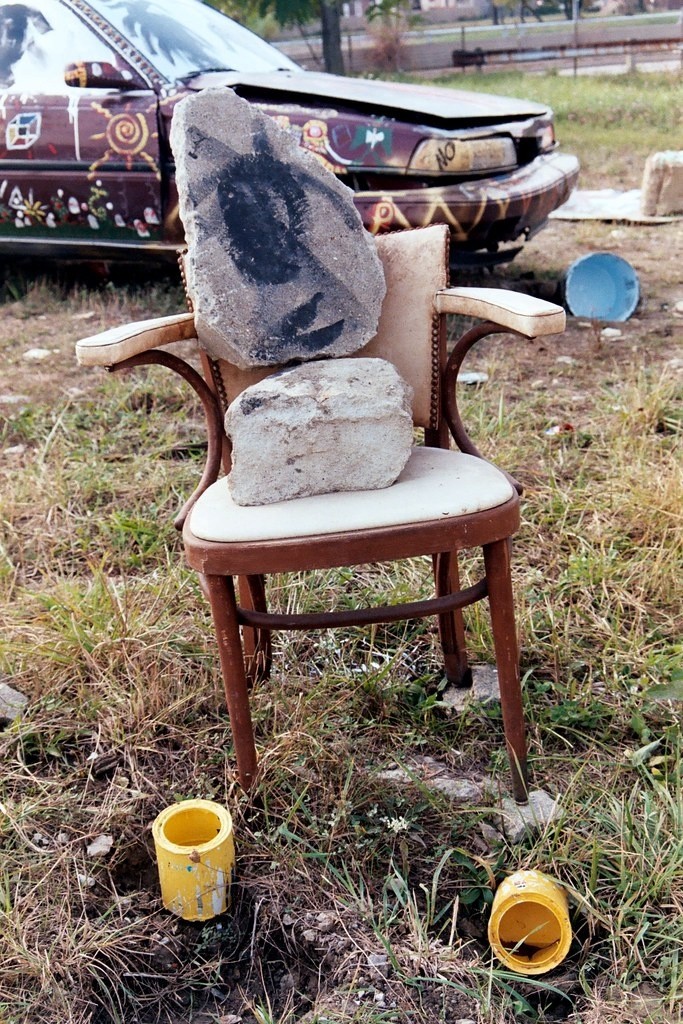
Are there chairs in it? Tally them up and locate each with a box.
[75,224,566,809]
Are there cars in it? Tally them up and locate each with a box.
[0,0,582,268]
